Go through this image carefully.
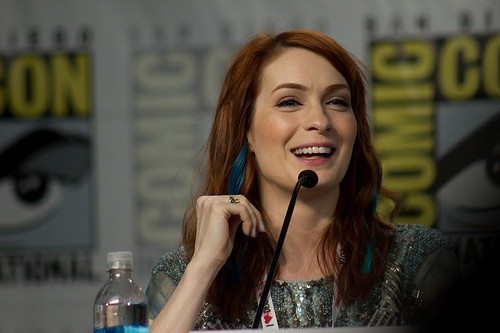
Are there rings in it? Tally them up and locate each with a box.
[229,196,240,204]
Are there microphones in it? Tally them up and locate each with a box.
[252,169,318,329]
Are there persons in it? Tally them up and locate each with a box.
[145,29,459,332]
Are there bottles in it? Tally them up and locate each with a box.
[92,251,149,333]
